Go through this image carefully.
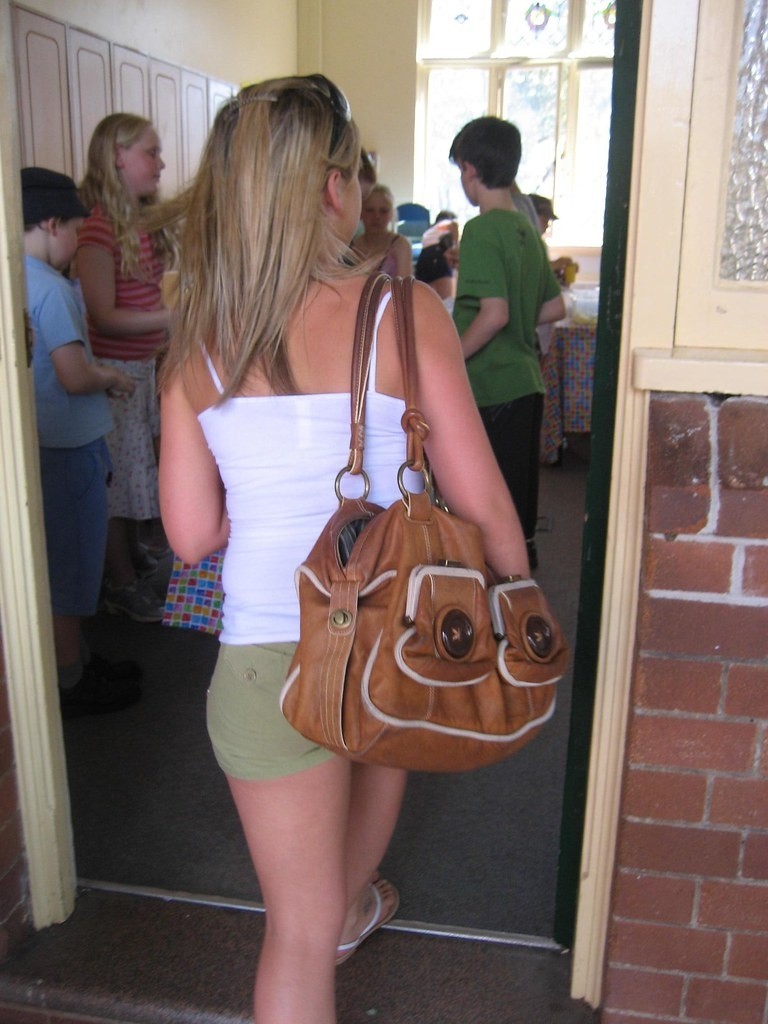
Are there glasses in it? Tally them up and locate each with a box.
[306,71,352,160]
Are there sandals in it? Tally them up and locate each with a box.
[333,880,399,966]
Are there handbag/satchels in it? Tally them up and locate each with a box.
[281,276,568,777]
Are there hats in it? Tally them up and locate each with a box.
[20,167,91,227]
[531,195,558,221]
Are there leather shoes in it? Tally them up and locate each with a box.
[59,668,145,717]
[83,656,143,686]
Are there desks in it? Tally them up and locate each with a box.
[537,316,596,465]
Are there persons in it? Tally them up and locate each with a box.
[107,69,538,1024]
[351,110,579,576]
[20,112,178,714]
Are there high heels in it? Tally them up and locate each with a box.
[105,574,168,623]
[134,553,160,577]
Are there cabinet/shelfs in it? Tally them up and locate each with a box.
[9,2,239,227]
